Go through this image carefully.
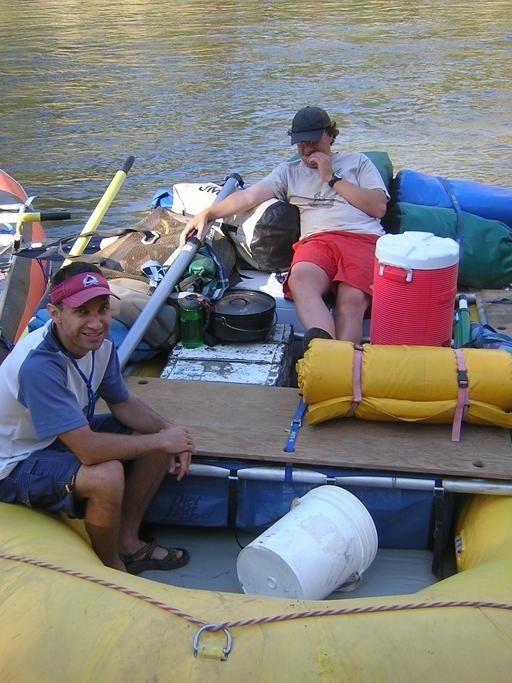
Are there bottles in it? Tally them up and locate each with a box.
[181,294,204,349]
[454,294,471,349]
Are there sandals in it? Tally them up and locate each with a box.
[122,543,189,574]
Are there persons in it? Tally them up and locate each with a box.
[180,107,391,352]
[0,262,195,575]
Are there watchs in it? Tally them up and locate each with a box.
[328,171,343,187]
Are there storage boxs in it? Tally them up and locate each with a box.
[159,324,294,386]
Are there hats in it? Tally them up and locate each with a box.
[48,272,120,307]
[292,106,331,145]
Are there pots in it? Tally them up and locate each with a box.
[208,289,276,342]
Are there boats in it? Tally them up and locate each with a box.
[0,149,51,364]
[0,188,512,683]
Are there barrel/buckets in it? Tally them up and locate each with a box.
[236,484,379,600]
[370,231,460,347]
